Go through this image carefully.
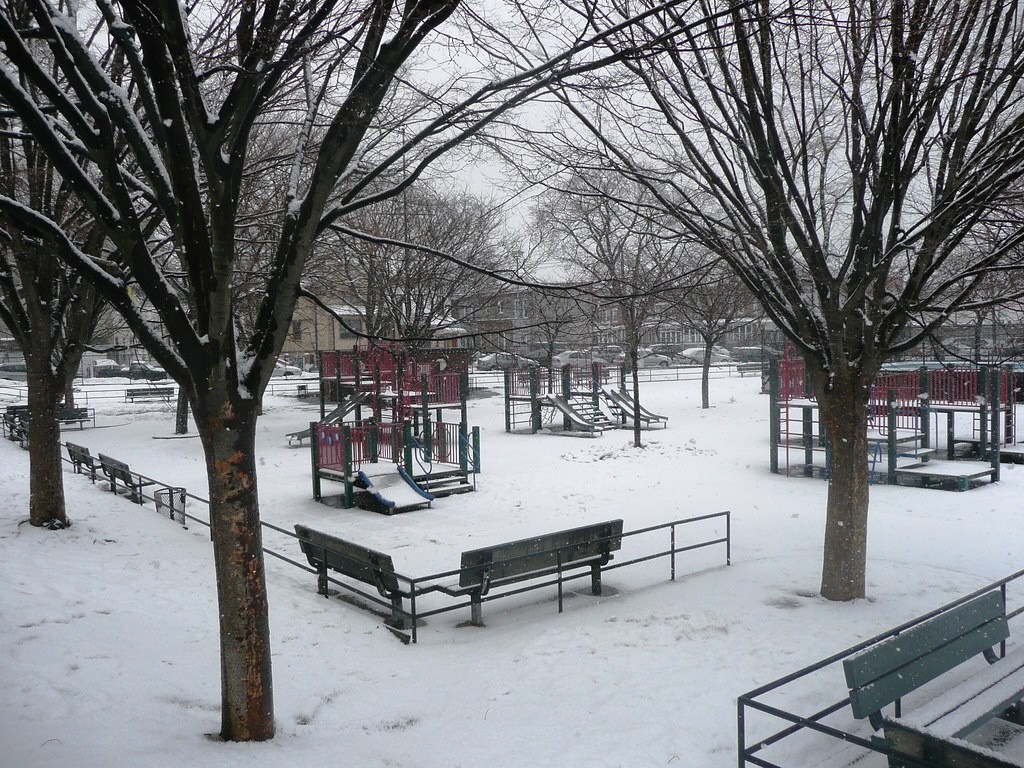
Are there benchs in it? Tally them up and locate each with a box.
[6,403,65,418]
[842,590,1024,748]
[65,441,102,484]
[98,453,155,503]
[18,415,30,445]
[2,413,22,441]
[58,408,91,430]
[293,523,428,631]
[736,364,770,378]
[573,369,612,385]
[434,519,624,628]
[126,387,174,403]
[517,372,555,388]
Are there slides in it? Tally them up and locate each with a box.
[285,390,372,440]
[603,388,669,423]
[358,467,435,509]
[547,393,605,431]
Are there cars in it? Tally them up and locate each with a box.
[552,351,610,367]
[477,352,541,370]
[87,359,121,373]
[734,341,795,362]
[674,348,733,365]
[526,347,570,367]
[648,344,685,358]
[120,361,167,380]
[938,336,1024,358]
[586,346,626,362]
[703,345,731,357]
[614,349,672,368]
[241,357,303,378]
